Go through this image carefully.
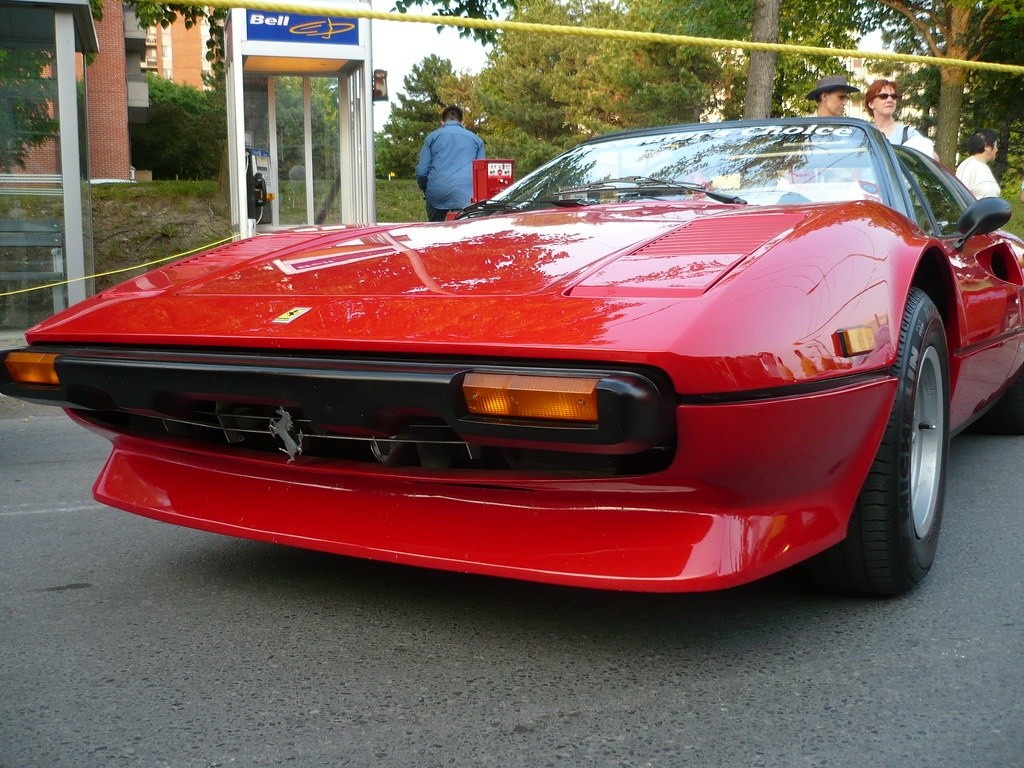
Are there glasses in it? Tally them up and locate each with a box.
[827,92,849,103]
[872,94,899,100]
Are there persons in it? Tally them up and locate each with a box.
[864,79,938,197]
[955,129,1001,200]
[803,76,862,117]
[415,104,490,223]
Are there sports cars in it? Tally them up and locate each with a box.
[1,118,1024,602]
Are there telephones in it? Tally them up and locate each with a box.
[245,147,276,208]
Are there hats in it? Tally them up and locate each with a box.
[805,77,860,100]
